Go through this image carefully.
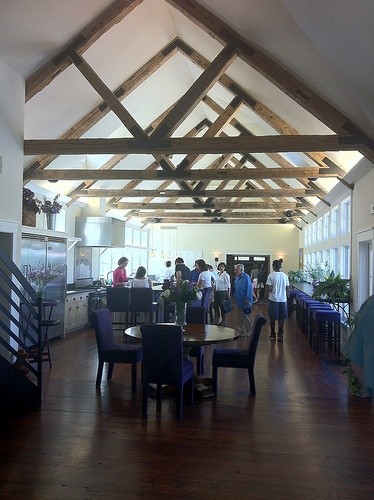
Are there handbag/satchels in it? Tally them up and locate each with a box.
[222,297,233,312]
[243,303,252,315]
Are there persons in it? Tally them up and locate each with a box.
[265,260,291,342]
[114,257,263,338]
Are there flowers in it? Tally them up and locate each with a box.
[23,188,41,214]
[161,279,202,308]
[37,194,62,214]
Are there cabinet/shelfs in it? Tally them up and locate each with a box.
[64,291,105,337]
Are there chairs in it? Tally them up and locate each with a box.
[91,287,266,426]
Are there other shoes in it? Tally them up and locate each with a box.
[218,323,228,327]
[246,332,251,337]
[253,300,258,303]
[240,332,247,337]
[269,333,276,339]
[277,334,283,340]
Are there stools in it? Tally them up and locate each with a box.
[288,289,340,361]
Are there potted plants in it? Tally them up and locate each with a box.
[312,270,350,302]
[336,311,370,397]
[288,271,307,284]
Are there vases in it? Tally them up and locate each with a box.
[46,213,56,230]
[173,302,188,326]
[22,209,36,228]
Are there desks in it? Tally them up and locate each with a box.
[125,322,240,360]
[290,281,350,318]
